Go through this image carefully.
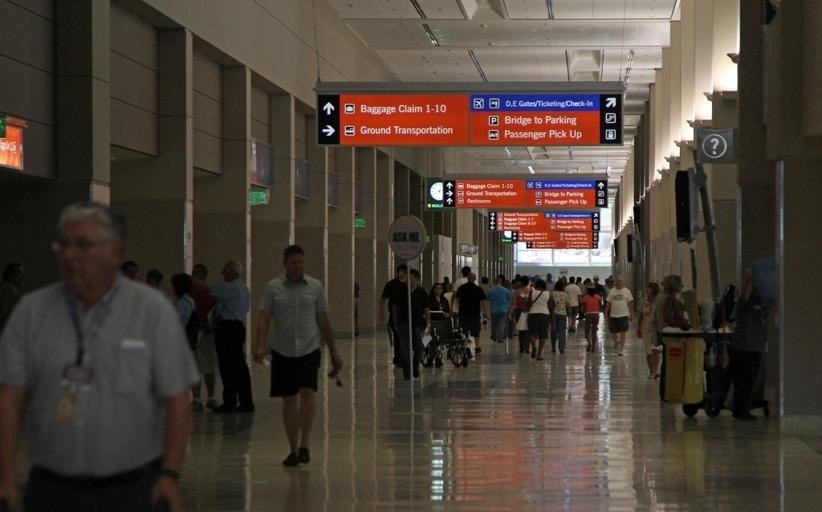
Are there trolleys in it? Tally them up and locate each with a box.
[646,327,774,422]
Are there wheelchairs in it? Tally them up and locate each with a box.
[419,310,472,370]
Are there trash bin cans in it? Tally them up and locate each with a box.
[661,333,705,403]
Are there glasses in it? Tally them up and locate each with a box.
[331,369,345,387]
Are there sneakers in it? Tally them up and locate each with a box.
[206,399,222,410]
[586,344,624,356]
[191,398,203,411]
[233,403,257,413]
[731,412,760,422]
[404,371,420,382]
[520,347,565,361]
[281,451,300,466]
[213,402,235,412]
[648,369,661,379]
[298,447,310,464]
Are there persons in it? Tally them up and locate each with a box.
[355,279,360,339]
[0,262,25,332]
[118,258,259,415]
[0,197,208,512]
[727,271,774,422]
[376,259,691,384]
[252,239,343,468]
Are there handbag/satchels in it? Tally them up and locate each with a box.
[524,299,535,310]
[516,310,529,331]
[637,314,644,338]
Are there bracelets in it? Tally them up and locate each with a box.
[159,466,178,481]
